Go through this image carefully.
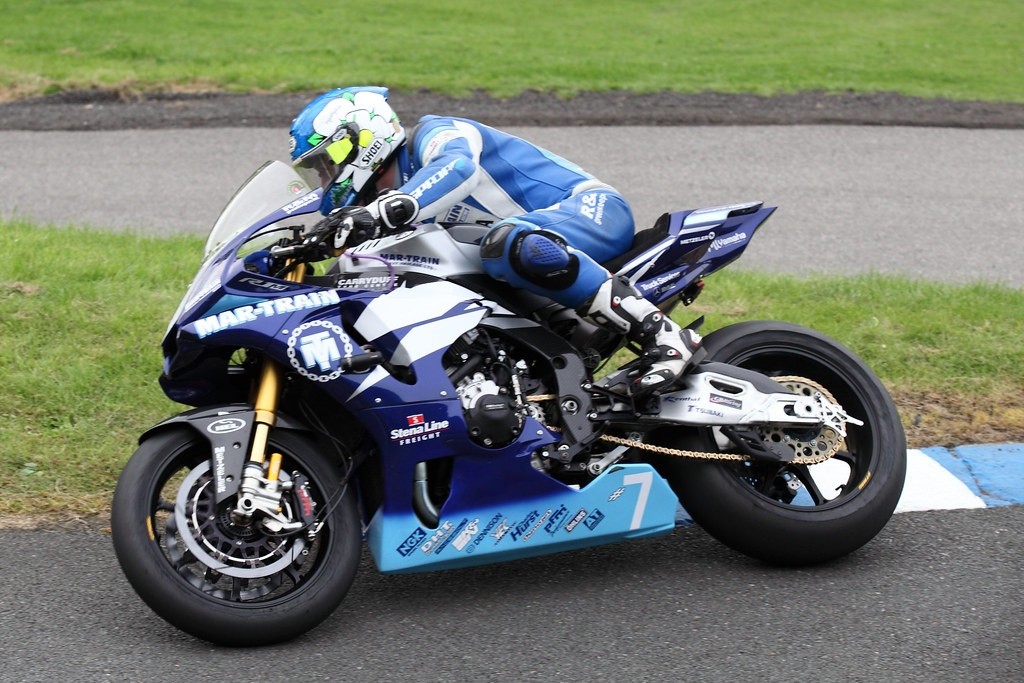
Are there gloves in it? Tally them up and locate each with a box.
[303,206,375,258]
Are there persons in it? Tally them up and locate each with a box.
[290,84,702,392]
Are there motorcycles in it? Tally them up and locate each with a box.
[110,159,905,649]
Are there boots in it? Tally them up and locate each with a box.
[577,272,703,393]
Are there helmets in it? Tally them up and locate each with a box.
[288,85,406,217]
